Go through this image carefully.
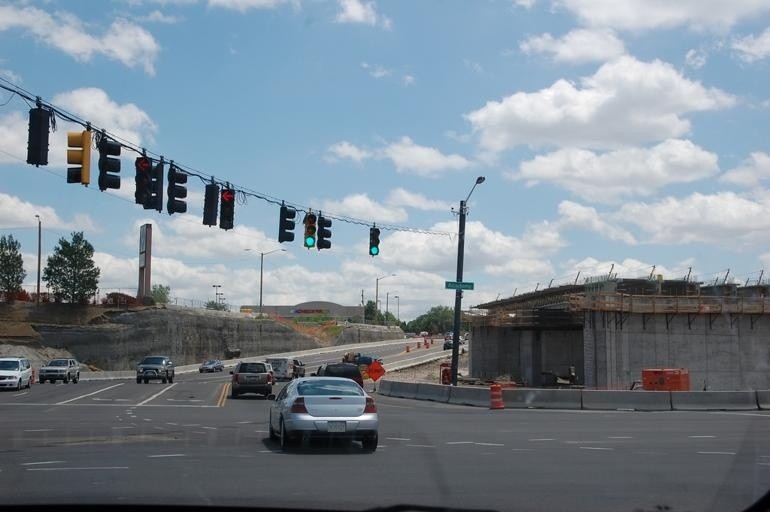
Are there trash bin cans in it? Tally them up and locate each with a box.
[440,363,452,385]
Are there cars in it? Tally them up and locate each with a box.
[444,331,470,351]
[199,359,224,374]
[229,362,242,373]
[266,375,380,454]
[265,363,276,385]
[293,360,306,378]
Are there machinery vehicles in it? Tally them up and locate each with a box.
[438,362,464,387]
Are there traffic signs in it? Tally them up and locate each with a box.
[445,280,475,291]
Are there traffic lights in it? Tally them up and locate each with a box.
[219,187,236,231]
[316,215,333,251]
[369,227,381,257]
[278,206,296,243]
[98,137,121,192]
[166,167,188,216]
[142,163,164,213]
[134,156,153,205]
[65,128,91,187]
[304,212,317,248]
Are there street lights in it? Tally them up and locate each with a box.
[394,296,399,326]
[244,248,288,313]
[450,174,486,385]
[377,299,381,313]
[35,213,42,303]
[213,285,227,306]
[375,274,396,311]
[386,289,398,322]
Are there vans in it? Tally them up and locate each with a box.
[266,356,294,381]
[0,358,32,390]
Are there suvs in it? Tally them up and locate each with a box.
[311,362,363,391]
[230,360,272,398]
[136,356,175,385]
[39,357,80,384]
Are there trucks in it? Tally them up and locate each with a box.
[341,351,384,366]
[631,367,690,392]
